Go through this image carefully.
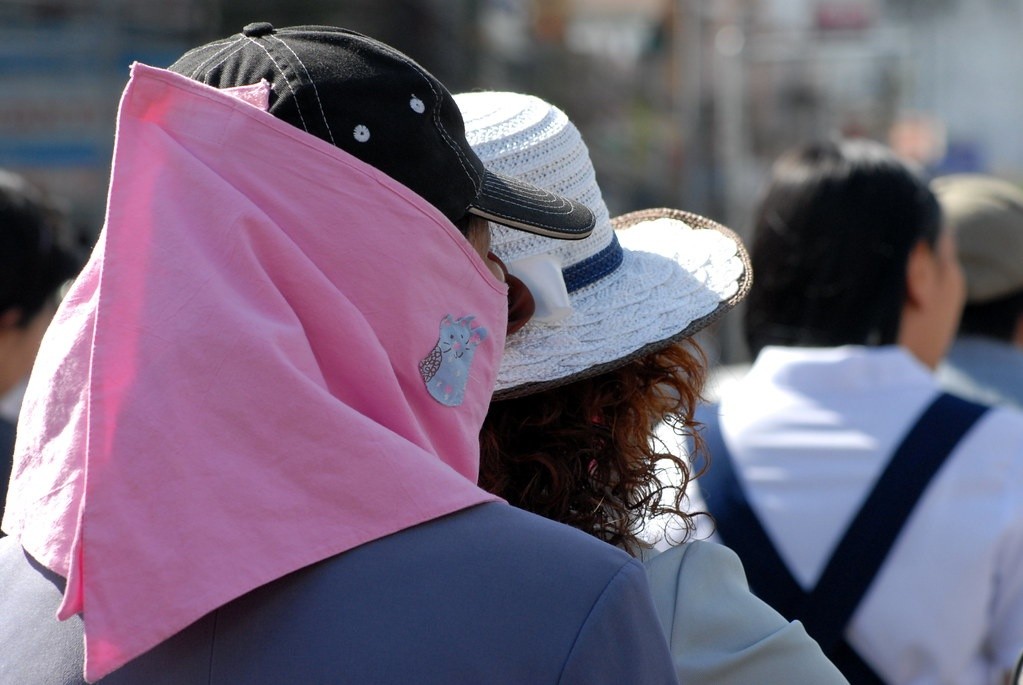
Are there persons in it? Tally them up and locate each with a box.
[1,22,681,685]
[454,91,852,685]
[628,123,1023,685]
[927,172,1023,409]
[0,169,84,520]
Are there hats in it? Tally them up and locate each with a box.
[162,21,597,240]
[926,172,1023,302]
[447,90,754,403]
